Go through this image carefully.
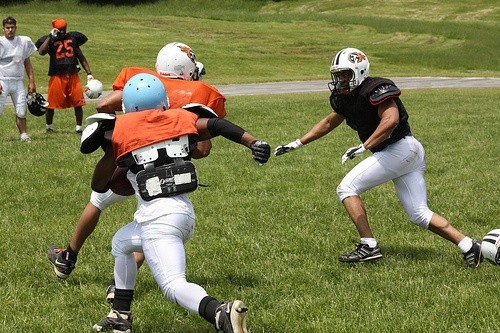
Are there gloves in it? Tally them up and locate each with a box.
[251,139,271,166]
[274,138,303,157]
[341,143,367,165]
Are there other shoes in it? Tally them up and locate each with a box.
[20,136,31,143]
[76,130,83,135]
[46,128,53,132]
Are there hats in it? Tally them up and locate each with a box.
[52,18,67,30]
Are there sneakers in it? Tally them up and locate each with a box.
[339,241,383,263]
[106,285,117,303]
[47,246,75,279]
[215,300,248,333]
[462,239,483,270]
[92,309,132,333]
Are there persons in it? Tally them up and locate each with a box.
[274,48,482,270]
[46,42,228,302]
[35,19,95,133]
[80,72,271,333]
[0,16,38,143]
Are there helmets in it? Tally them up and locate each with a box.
[122,73,168,114]
[85,79,103,99]
[155,42,196,79]
[26,92,49,117]
[481,229,500,266]
[328,48,369,107]
[196,61,206,81]
